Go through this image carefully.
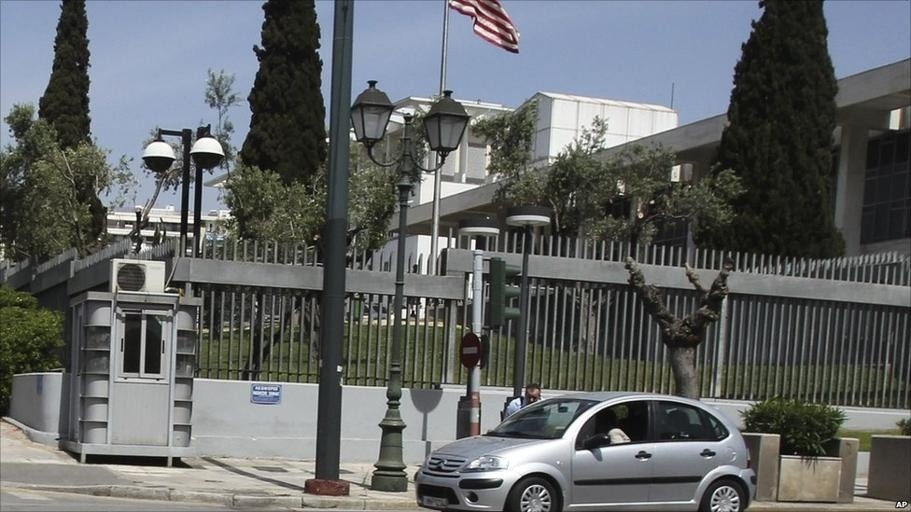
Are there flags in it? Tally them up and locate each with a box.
[449,0,521,57]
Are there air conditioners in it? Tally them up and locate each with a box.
[113,258,165,296]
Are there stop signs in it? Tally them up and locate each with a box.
[459,332,482,370]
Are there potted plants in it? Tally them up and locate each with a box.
[738,395,911,504]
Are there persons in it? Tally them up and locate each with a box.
[500,382,553,437]
[584,408,631,445]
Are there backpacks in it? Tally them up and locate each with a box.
[500,396,524,422]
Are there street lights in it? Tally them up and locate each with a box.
[142,124,225,297]
[507,206,550,396]
[349,79,471,492]
[459,218,501,249]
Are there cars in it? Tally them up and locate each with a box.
[413,391,757,512]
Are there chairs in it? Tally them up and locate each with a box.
[596,408,693,438]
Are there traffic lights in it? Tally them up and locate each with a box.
[489,258,523,328]
